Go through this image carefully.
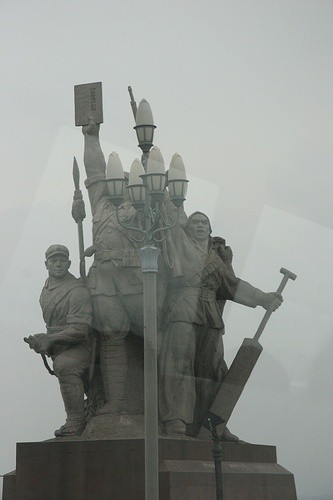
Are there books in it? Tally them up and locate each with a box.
[73,81,104,126]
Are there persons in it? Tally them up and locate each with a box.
[25,243,95,438]
[81,119,147,416]
[141,152,284,434]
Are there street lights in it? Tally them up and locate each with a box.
[106,98,191,500]
